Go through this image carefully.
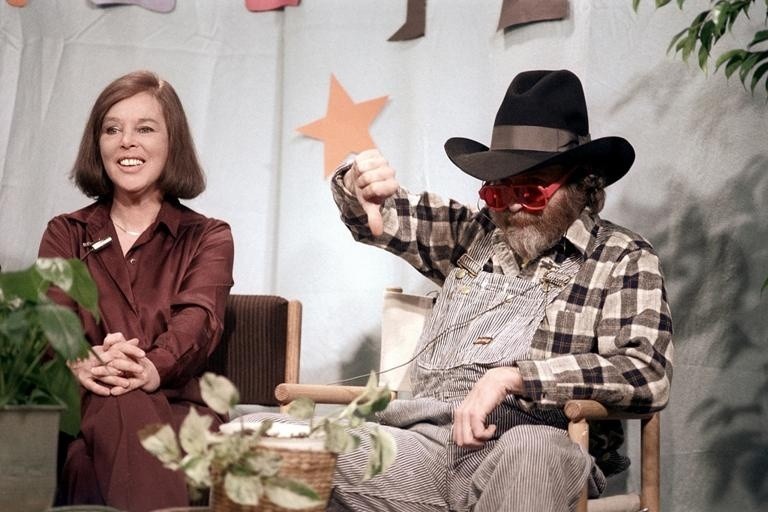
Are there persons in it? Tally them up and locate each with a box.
[329,68,675,511]
[38,70,233,512]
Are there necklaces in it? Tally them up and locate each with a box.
[112,222,142,236]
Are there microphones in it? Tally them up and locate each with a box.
[91,236,113,253]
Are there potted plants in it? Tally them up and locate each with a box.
[138,372,400,512]
[0,254,102,511]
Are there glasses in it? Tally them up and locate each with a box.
[478,170,572,212]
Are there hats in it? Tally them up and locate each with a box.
[443,70,636,189]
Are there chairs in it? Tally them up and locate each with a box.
[207,295,302,420]
[275,288,663,511]
